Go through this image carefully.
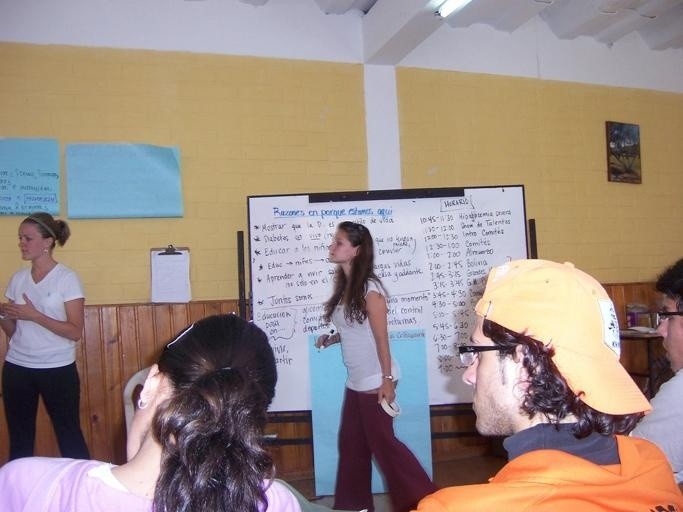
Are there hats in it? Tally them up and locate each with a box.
[474,258,654,416]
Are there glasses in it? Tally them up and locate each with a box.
[459,346,511,366]
[656,312,683,326]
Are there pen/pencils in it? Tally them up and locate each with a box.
[318,329,334,352]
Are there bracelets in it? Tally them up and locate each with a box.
[380,374,392,380]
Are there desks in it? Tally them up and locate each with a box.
[620,329,673,399]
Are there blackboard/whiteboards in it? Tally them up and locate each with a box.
[246,184,531,412]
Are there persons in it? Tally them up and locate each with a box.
[0,313,302,512]
[0,211,94,463]
[413,258,683,512]
[314,221,437,512]
[620,262,682,493]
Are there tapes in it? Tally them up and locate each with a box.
[379,397,400,418]
